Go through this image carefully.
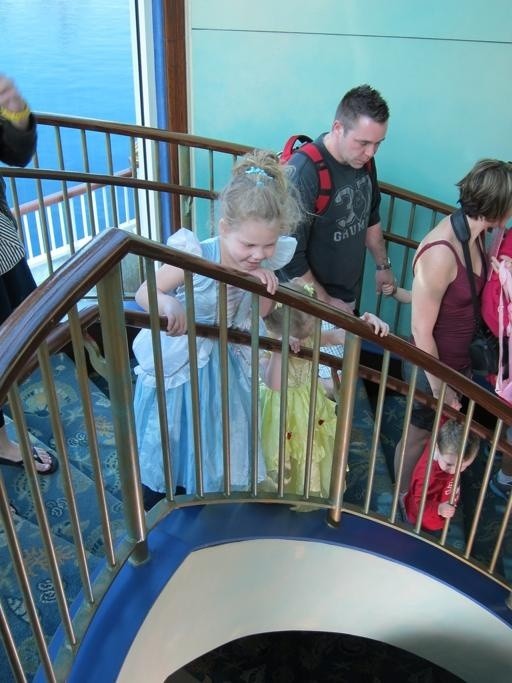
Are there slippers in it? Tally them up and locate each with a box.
[0,440,61,476]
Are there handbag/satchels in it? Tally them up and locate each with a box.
[470,326,504,378]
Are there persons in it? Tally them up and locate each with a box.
[125,148,299,499]
[402,411,483,542]
[490,423,512,504]
[259,278,394,496]
[479,221,512,376]
[390,149,512,521]
[381,274,416,304]
[281,73,400,400]
[0,70,62,479]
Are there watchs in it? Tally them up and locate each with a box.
[376,255,392,271]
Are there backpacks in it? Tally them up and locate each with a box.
[275,133,374,217]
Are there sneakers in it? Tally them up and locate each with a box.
[484,439,503,458]
[489,469,511,504]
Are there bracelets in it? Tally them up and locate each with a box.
[0,104,31,121]
